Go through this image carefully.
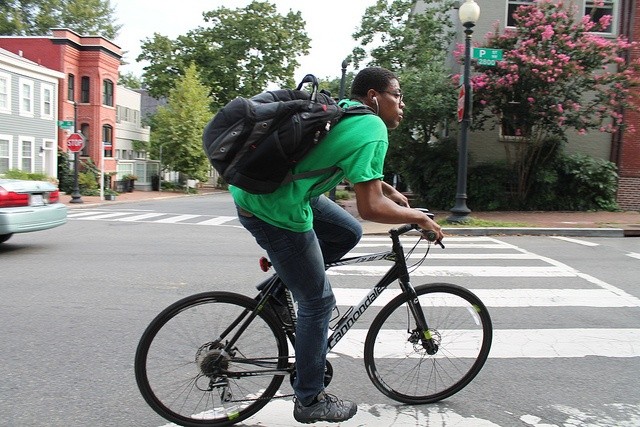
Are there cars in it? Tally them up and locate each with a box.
[0,177,67,242]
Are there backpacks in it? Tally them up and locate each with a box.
[201,73,379,194]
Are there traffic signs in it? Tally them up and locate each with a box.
[57,121,73,128]
[473,48,503,66]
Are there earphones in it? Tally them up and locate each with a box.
[372,96,379,106]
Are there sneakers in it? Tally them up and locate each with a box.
[271,287,296,328]
[294,387,358,423]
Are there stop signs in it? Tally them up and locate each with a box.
[67,133,84,152]
[458,84,465,122]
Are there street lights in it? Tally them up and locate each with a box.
[446,0,480,223]
[159,140,175,190]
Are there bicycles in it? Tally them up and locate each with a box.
[135,201,492,426]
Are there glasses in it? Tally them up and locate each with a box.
[374,88,404,102]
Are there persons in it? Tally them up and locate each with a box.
[227,66,445,424]
